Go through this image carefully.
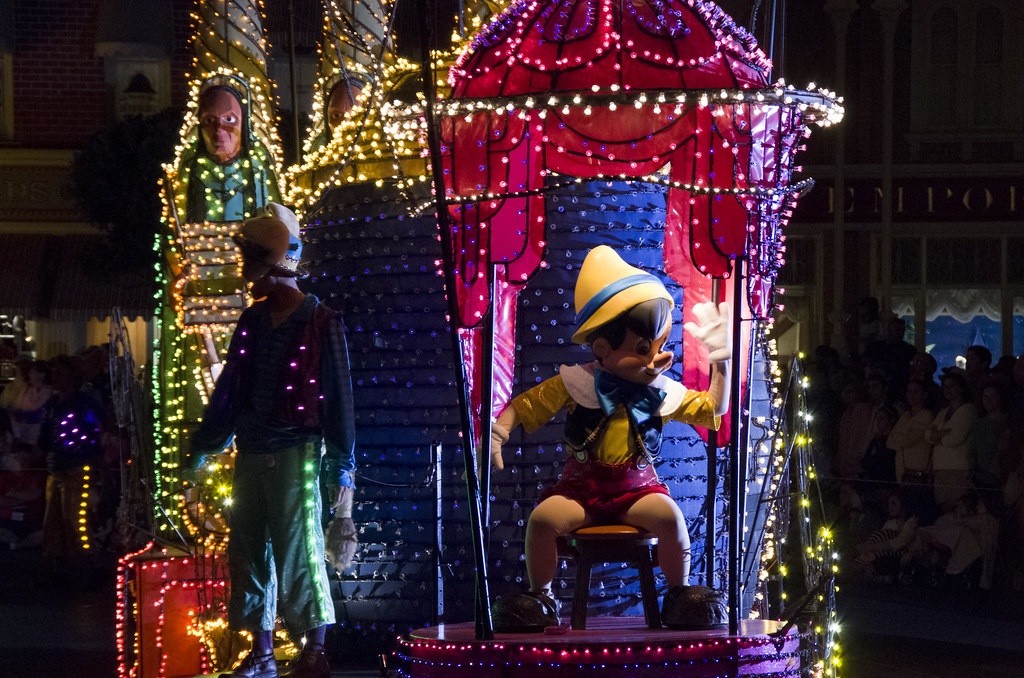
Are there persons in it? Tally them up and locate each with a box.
[461,244,731,633]
[296,80,369,193]
[181,217,357,678]
[183,84,284,220]
[778,320,1024,608]
[1,343,132,590]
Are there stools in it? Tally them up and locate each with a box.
[571,523,662,630]
